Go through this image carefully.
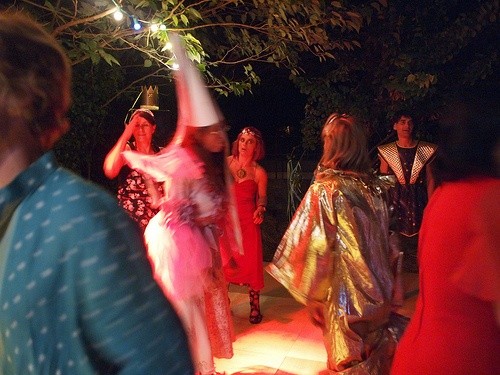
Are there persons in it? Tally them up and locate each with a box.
[266,111,500,375]
[0,12,195,375]
[105,39,267,375]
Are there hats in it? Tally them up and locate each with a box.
[170,34,225,127]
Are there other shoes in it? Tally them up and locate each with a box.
[249,290,262,324]
